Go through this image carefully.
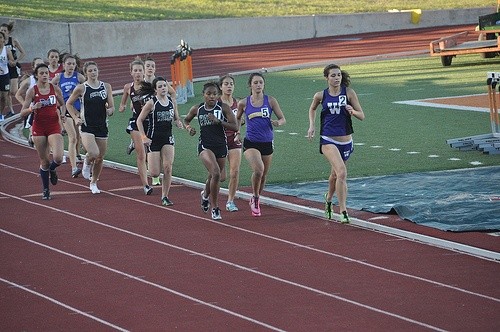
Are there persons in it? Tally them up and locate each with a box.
[0,22,100,181]
[136,76,182,206]
[66,61,115,194]
[307,63,365,223]
[20,63,66,200]
[184,82,239,220]
[234,72,287,217]
[218,73,245,211]
[119,56,177,195]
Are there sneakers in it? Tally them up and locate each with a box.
[340,210,351,223]
[28,134,34,147]
[6,111,14,117]
[42,188,52,200]
[0,114,4,122]
[126,138,136,155]
[77,155,83,164]
[143,184,153,196]
[90,183,100,194]
[50,168,58,185]
[162,197,173,206]
[226,201,238,211]
[82,158,91,179]
[71,167,82,178]
[200,190,210,214]
[152,177,161,186]
[249,196,261,216]
[324,193,335,220]
[211,208,222,220]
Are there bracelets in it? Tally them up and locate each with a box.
[29,106,33,112]
[186,124,191,131]
[234,132,241,138]
[219,119,222,124]
